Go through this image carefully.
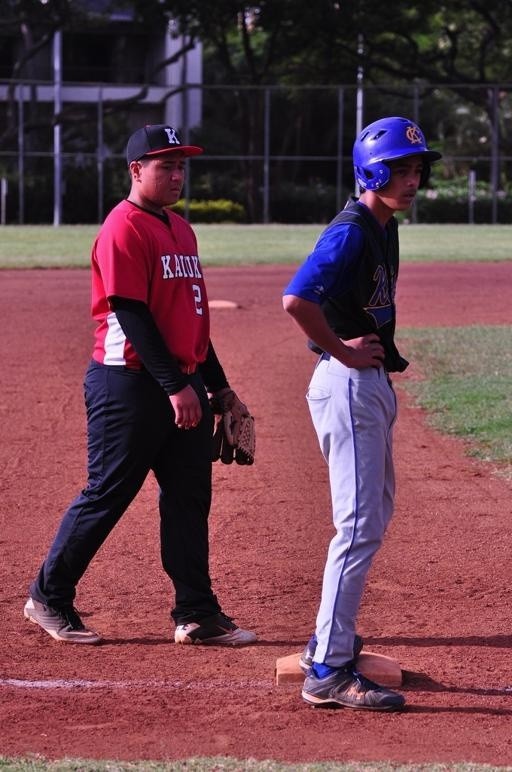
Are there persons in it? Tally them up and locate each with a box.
[282,116,442,711]
[23,124,258,645]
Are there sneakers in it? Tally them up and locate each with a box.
[23,597,101,645]
[174,610,257,646]
[299,632,363,672]
[302,666,404,711]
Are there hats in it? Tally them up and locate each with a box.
[127,125,202,165]
[353,117,441,190]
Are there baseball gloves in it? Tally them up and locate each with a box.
[208,390,256,466]
